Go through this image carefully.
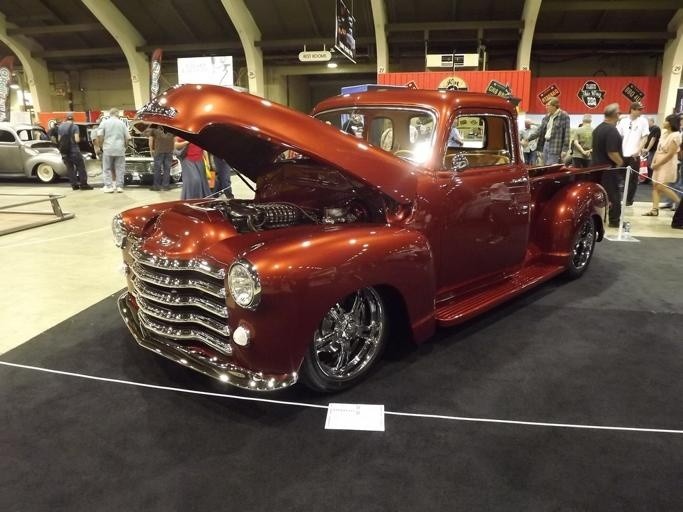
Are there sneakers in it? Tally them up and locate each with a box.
[610,220,629,228]
[149,184,171,192]
[659,201,680,212]
[70,184,94,190]
[104,187,115,193]
[116,186,123,193]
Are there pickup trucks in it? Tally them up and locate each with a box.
[114,89,613,403]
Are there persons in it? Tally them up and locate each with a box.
[174,136,213,200]
[58,115,94,191]
[518,97,683,230]
[448,118,463,148]
[47,123,59,144]
[342,113,363,139]
[208,151,234,200]
[90,125,117,190]
[143,126,174,191]
[97,107,131,193]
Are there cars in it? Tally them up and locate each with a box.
[0,122,81,185]
[113,119,179,188]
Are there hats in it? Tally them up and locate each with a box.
[629,101,646,110]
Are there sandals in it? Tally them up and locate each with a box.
[642,208,660,218]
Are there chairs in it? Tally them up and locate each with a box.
[390,144,510,169]
